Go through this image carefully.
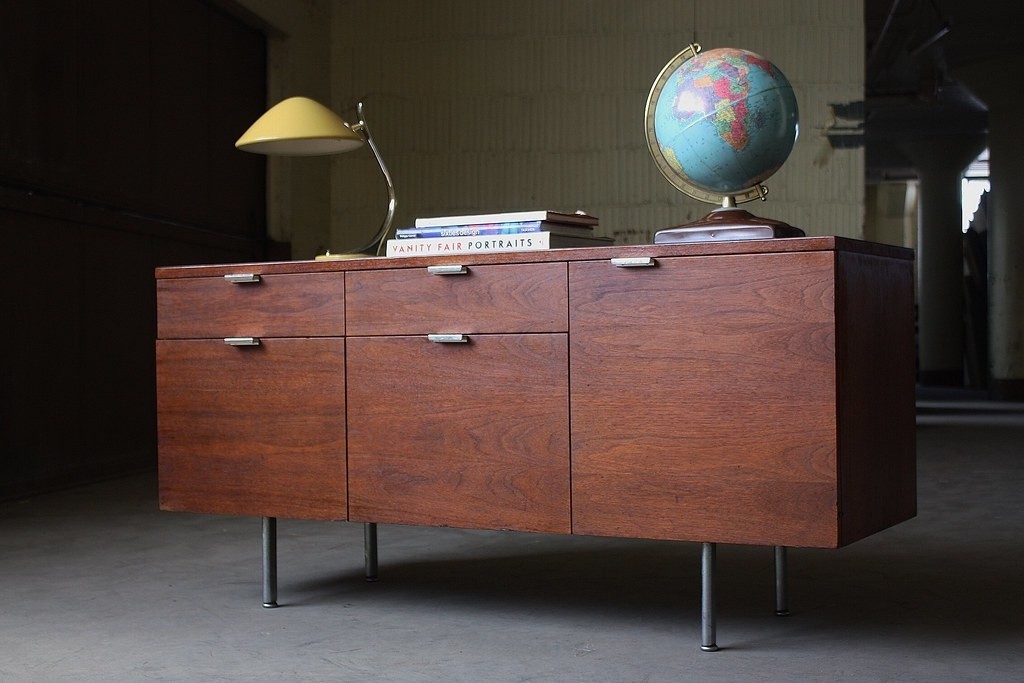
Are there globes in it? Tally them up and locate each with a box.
[643,41,807,243]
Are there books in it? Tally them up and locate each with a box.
[385,209,614,260]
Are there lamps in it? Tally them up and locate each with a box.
[234,94,396,263]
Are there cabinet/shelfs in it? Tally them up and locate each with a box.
[0,0,290,500]
[154,237,917,653]
